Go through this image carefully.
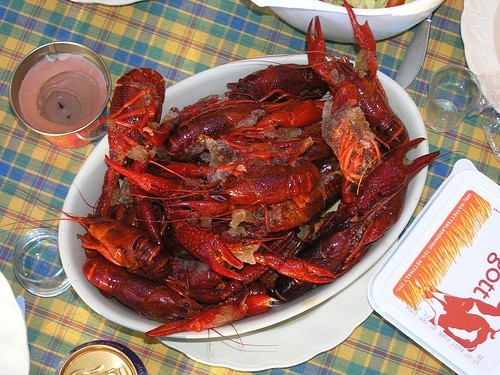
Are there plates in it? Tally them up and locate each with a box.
[460,0,499,116]
[1,271,31,375]
[157,238,402,371]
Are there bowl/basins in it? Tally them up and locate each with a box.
[57,53,431,341]
[9,42,112,149]
[249,0,445,44]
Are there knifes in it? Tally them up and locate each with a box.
[396,12,433,90]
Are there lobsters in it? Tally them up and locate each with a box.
[0,0,468,360]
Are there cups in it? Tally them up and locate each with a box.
[13,226,71,297]
[423,64,482,131]
